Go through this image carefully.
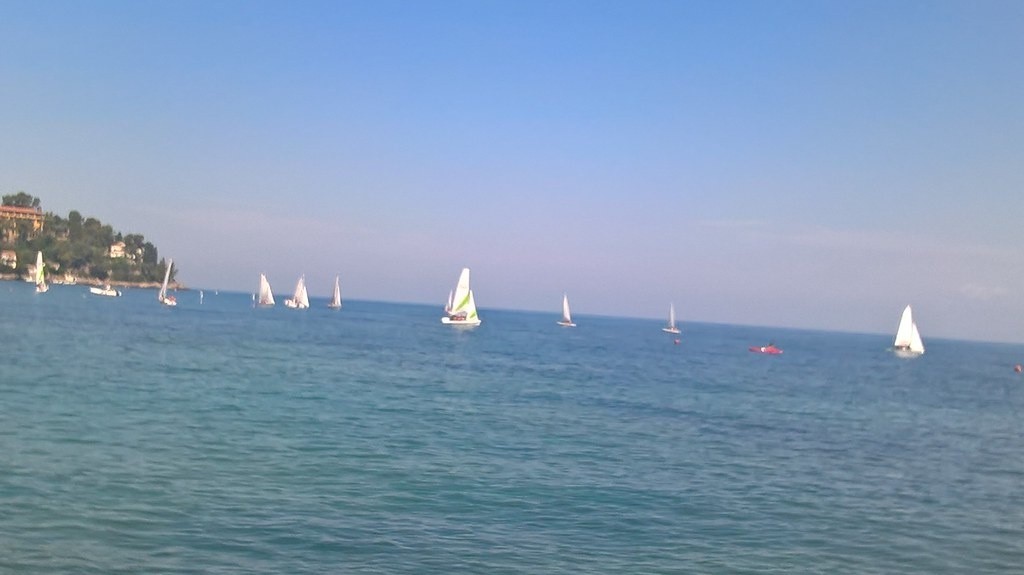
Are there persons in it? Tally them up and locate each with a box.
[675,338,680,343]
[104,280,111,291]
[168,295,177,302]
[761,343,772,352]
[450,315,465,320]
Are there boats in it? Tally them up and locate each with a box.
[89,286,123,298]
[749,345,784,356]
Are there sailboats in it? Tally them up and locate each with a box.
[157,258,178,308]
[34,251,50,294]
[557,294,578,328]
[440,268,483,327]
[252,273,276,308]
[283,274,309,308]
[893,304,926,360]
[663,301,683,334]
[327,273,343,310]
